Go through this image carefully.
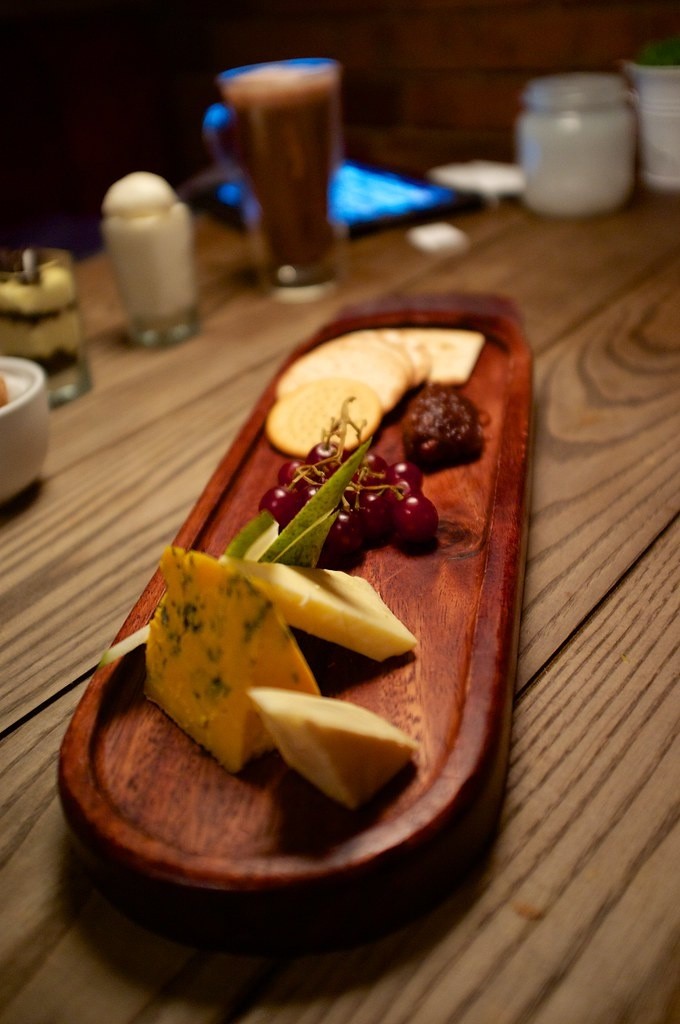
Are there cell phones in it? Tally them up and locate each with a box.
[189,155,484,239]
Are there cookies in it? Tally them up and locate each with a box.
[265,328,486,455]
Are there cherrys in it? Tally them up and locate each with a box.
[258,441,440,557]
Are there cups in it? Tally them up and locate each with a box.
[1,355,49,502]
[216,56,344,307]
[1,247,91,410]
[626,63,680,191]
[102,202,201,350]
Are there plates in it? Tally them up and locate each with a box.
[55,289,537,957]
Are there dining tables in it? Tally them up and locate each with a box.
[0,56,680,1021]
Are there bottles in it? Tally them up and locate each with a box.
[514,72,638,218]
